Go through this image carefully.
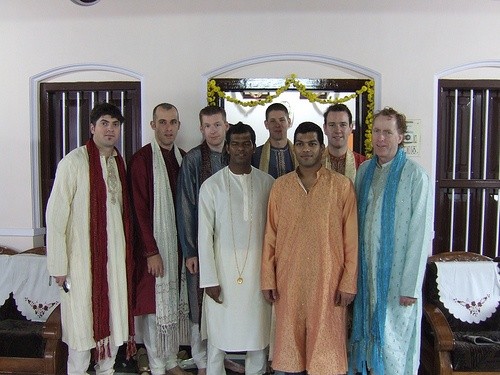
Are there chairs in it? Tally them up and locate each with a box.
[0,246,68,375]
[420,252,500,375]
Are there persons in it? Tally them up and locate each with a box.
[179,103,245,375]
[261,121,359,375]
[321,104,373,179]
[198,122,278,375]
[45,103,138,375]
[346,106,433,375]
[252,103,299,374]
[127,103,193,375]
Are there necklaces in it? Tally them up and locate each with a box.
[228,164,254,284]
